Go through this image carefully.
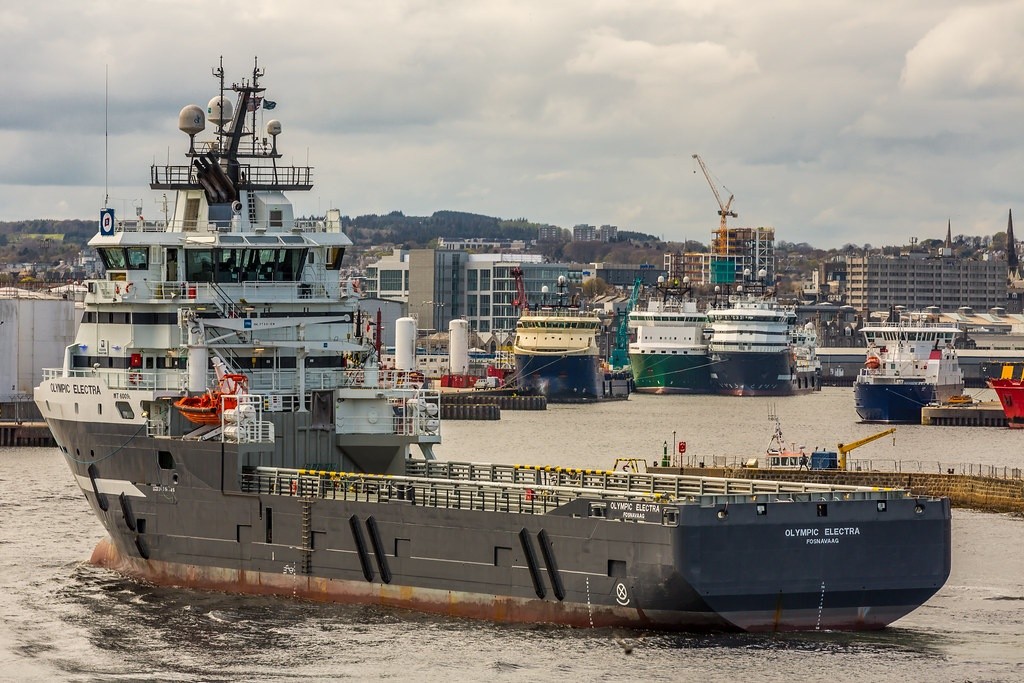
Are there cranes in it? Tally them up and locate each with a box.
[692,153,739,256]
[837,426,896,470]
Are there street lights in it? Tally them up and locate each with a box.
[422,300,432,354]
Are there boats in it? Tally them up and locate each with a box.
[624,290,822,396]
[763,400,812,471]
[853,316,966,425]
[513,304,606,403]
[31,51,954,638]
[984,364,1024,429]
[173,375,250,425]
